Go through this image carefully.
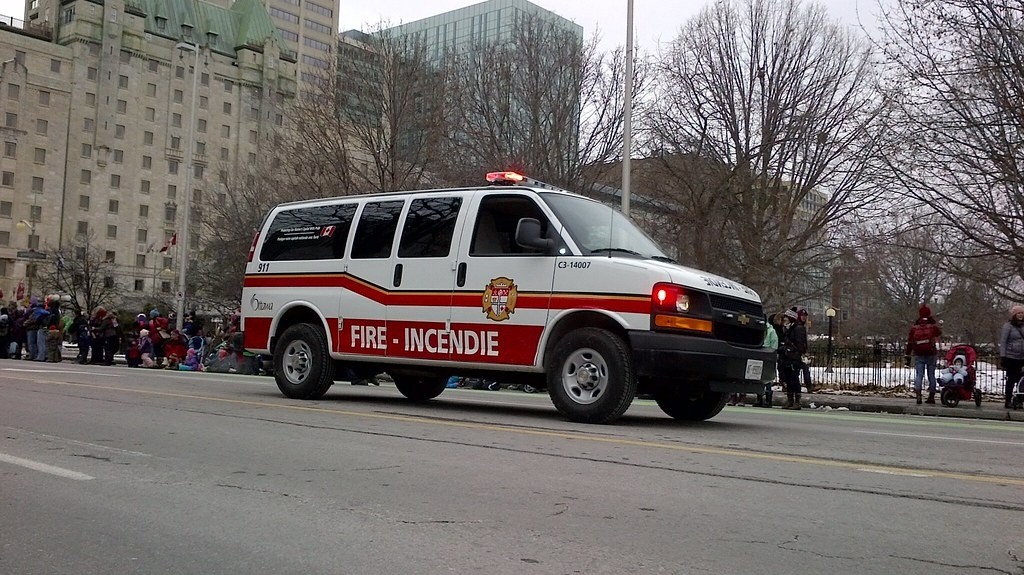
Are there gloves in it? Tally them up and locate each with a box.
[903,356,912,366]
[1000,357,1006,368]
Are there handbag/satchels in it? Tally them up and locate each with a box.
[800,355,815,364]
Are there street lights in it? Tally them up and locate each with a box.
[823,307,838,371]
[13,193,47,295]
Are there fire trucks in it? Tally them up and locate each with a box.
[240,167,775,423]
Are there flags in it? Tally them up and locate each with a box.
[159,233,177,253]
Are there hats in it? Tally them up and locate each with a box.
[1011,306,1024,317]
[919,306,931,318]
[140,329,150,338]
[784,307,798,320]
[150,309,159,318]
[800,309,808,316]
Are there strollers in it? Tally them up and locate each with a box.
[936,344,985,406]
[1012,375,1024,407]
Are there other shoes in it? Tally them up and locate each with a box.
[1005,401,1015,408]
[937,378,945,387]
[0,357,62,363]
[70,358,116,366]
[807,383,815,393]
[957,378,962,384]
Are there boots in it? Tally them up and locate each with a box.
[782,395,801,410]
[753,390,773,408]
[924,393,935,404]
[917,392,922,404]
[727,392,746,407]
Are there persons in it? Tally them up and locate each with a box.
[0,288,120,366]
[1000,305,1024,410]
[938,354,967,384]
[124,306,240,373]
[727,306,822,410]
[904,303,942,405]
